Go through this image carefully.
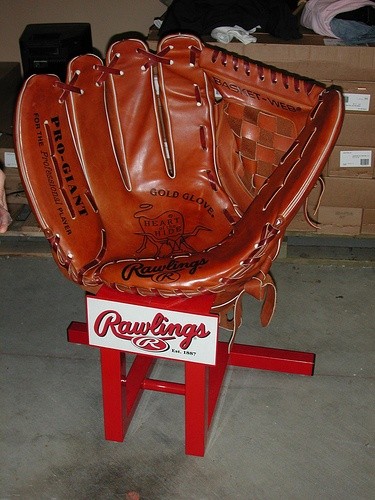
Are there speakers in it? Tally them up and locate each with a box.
[0,63,19,135]
[19,24,92,74]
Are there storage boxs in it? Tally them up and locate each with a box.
[197,32,374,238]
[0,61,22,112]
[0,101,32,203]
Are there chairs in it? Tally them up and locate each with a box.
[14,32,345,457]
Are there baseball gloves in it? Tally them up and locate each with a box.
[16,31,345,357]
